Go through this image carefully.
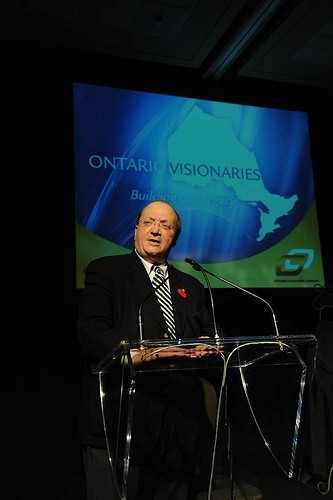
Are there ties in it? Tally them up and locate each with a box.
[151,267,176,340]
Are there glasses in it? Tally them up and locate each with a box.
[138,219,177,230]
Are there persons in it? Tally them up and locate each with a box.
[83,197,225,479]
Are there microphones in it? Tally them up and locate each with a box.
[185,258,219,339]
[137,264,175,340]
[192,265,280,336]
